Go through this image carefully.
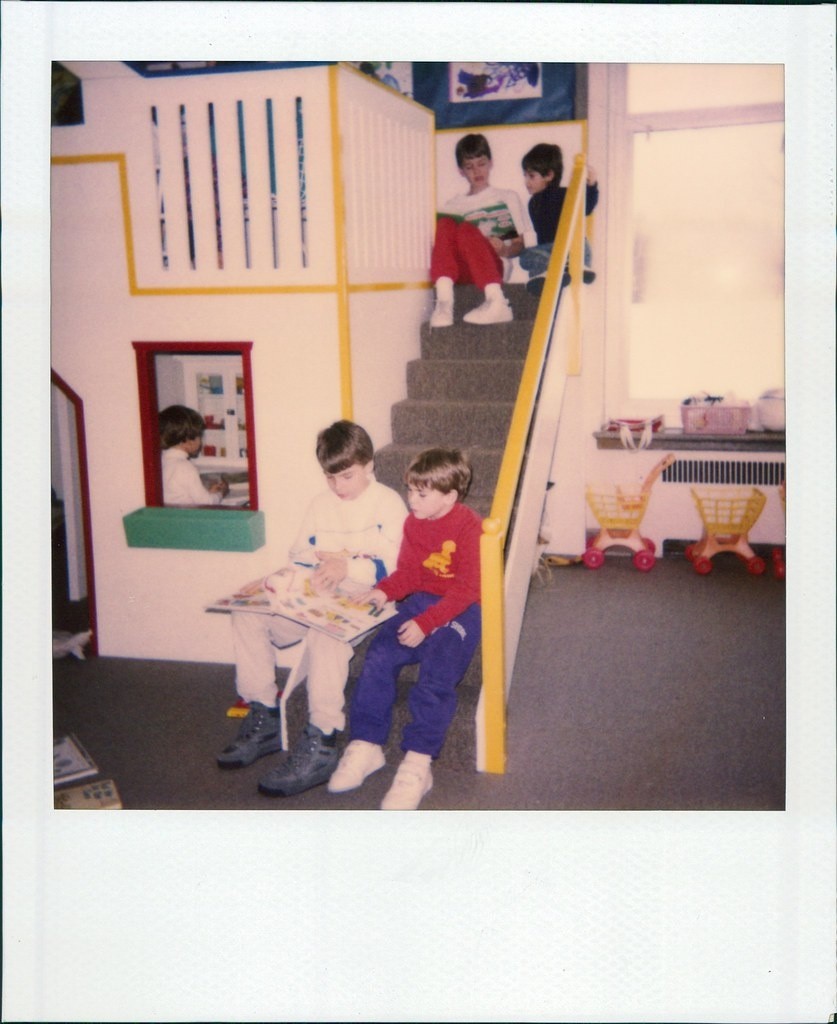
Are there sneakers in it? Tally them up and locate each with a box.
[256,725,338,795]
[380,760,433,810]
[217,701,281,770]
[462,297,515,324]
[428,297,455,327]
[584,265,596,284]
[525,270,571,295]
[325,739,385,792]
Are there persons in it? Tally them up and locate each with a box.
[517,142,600,294]
[430,133,529,328]
[158,404,229,506]
[216,420,410,797]
[327,447,482,810]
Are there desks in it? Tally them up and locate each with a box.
[592,429,786,453]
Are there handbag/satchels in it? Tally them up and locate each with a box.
[602,416,664,454]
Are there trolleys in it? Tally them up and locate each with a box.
[772,482,786,578]
[582,454,675,571]
[685,489,765,574]
